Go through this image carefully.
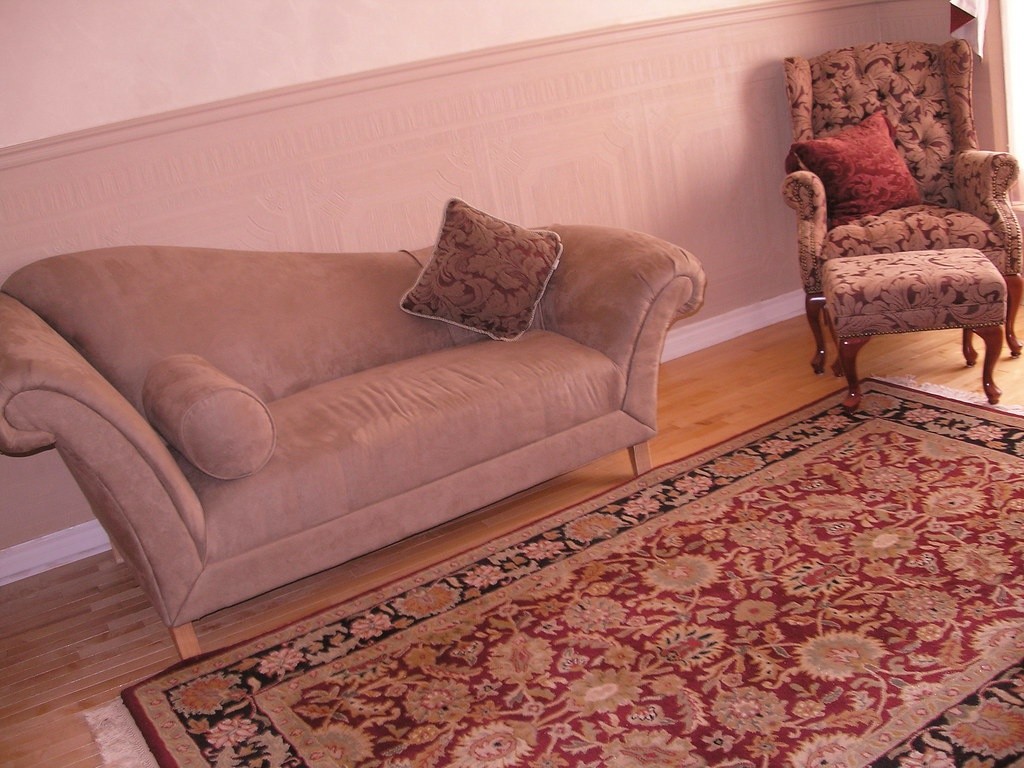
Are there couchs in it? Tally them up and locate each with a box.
[780,29,1023,374]
[0,224,706,664]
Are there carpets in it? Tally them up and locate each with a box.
[82,378,1024,768]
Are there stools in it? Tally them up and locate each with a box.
[819,248,1008,410]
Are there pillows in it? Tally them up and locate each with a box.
[143,353,276,480]
[399,196,564,340]
[793,106,923,231]
